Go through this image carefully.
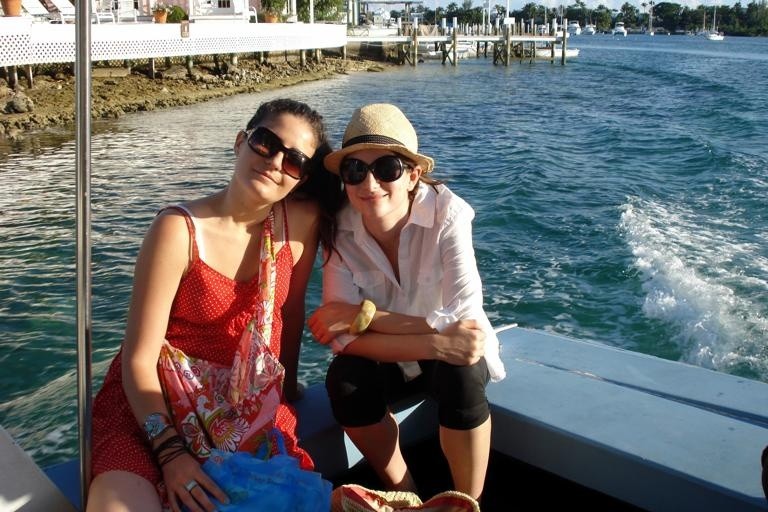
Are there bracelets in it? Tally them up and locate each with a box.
[346,299,378,336]
[139,411,173,441]
[152,434,187,465]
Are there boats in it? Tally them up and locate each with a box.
[408,41,494,58]
[569,20,581,35]
[706,33,725,41]
[585,27,595,35]
[556,27,570,38]
[517,45,580,57]
[614,21,626,34]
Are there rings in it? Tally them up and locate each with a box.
[185,481,197,489]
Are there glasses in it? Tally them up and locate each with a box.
[245,125,312,180]
[339,155,408,184]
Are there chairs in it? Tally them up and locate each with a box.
[92,0,138,25]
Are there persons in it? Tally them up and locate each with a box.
[303,102,506,509]
[82,100,336,512]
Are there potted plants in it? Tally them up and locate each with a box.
[260,0,286,23]
[150,2,171,25]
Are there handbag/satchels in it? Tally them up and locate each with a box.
[333,484,481,512]
[187,447,333,512]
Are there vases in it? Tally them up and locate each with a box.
[1,1,23,18]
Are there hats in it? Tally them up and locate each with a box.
[323,103,434,176]
